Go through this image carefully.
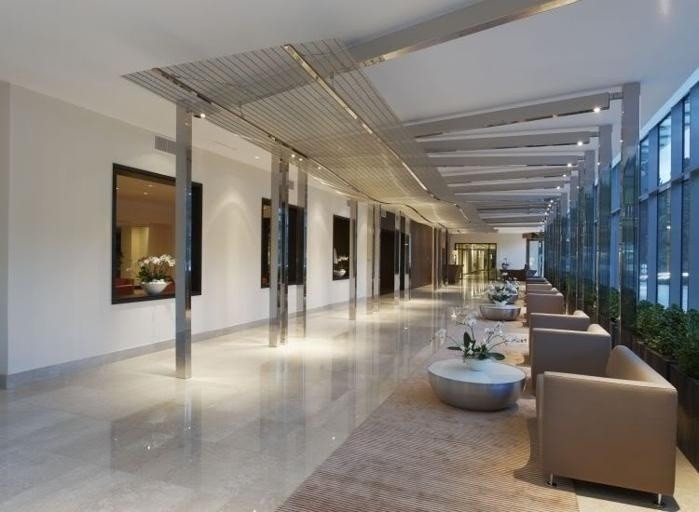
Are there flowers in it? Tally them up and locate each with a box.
[126,254,176,282]
[435,311,528,360]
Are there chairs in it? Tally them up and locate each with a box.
[525,277,678,509]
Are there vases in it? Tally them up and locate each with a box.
[145,281,168,296]
[467,358,491,371]
